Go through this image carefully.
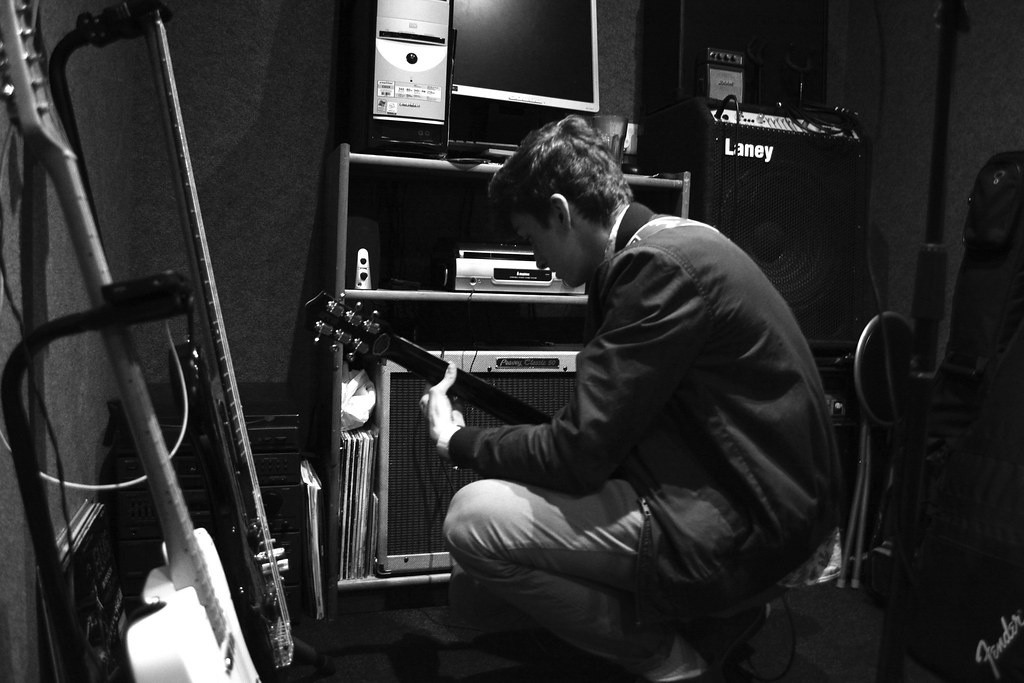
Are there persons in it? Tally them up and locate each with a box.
[419,111,839,683]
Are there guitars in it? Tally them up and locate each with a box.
[306,291,552,424]
[1,0,298,683]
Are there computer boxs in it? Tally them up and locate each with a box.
[332,0,453,159]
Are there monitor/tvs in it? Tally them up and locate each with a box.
[451,0,600,113]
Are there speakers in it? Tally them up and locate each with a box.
[363,344,585,575]
[636,0,875,350]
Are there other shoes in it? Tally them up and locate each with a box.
[669,602,767,680]
